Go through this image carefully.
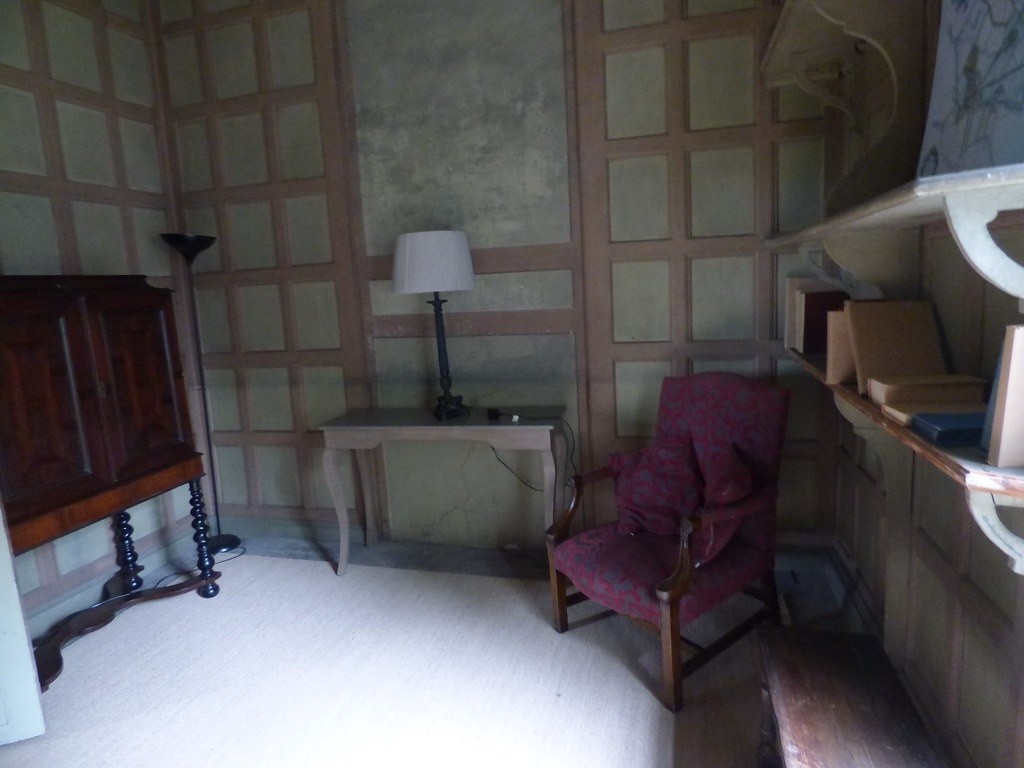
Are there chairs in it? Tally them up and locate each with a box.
[543,370,789,714]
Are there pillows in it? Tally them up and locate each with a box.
[685,440,780,571]
[608,430,696,533]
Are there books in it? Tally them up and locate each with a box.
[778,272,953,395]
[867,372,992,442]
[980,324,1024,469]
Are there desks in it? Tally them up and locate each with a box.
[318,405,570,576]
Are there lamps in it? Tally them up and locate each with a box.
[160,232,241,554]
[393,230,474,419]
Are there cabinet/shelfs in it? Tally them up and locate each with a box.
[756,163,1024,767]
